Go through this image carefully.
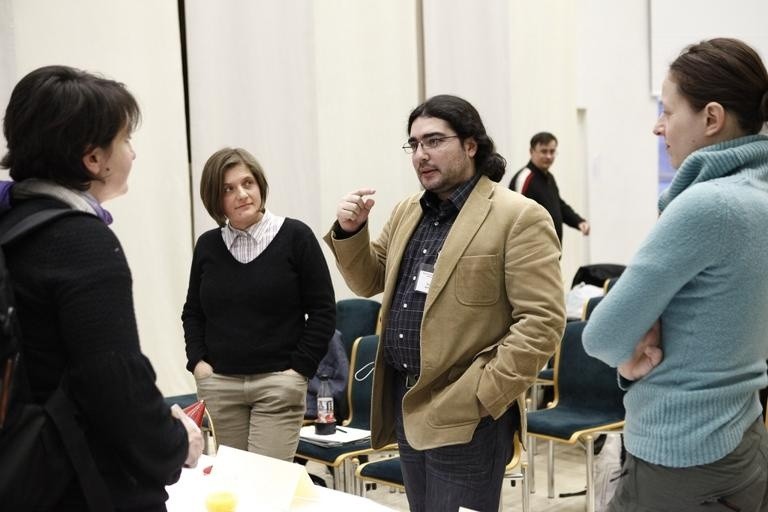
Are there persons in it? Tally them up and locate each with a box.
[319,94,568,512]
[0,66,208,512]
[181,147,338,461]
[577,37,768,512]
[301,327,351,420]
[507,132,592,261]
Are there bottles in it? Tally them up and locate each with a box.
[316,374,335,420]
[202,429,217,456]
[174,398,207,469]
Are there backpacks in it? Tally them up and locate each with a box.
[0,207,113,512]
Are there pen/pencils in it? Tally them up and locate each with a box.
[336,428,347,433]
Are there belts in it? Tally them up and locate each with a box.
[400,369,419,390]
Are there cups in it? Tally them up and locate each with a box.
[314,419,337,435]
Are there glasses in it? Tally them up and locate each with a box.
[402,135,460,154]
